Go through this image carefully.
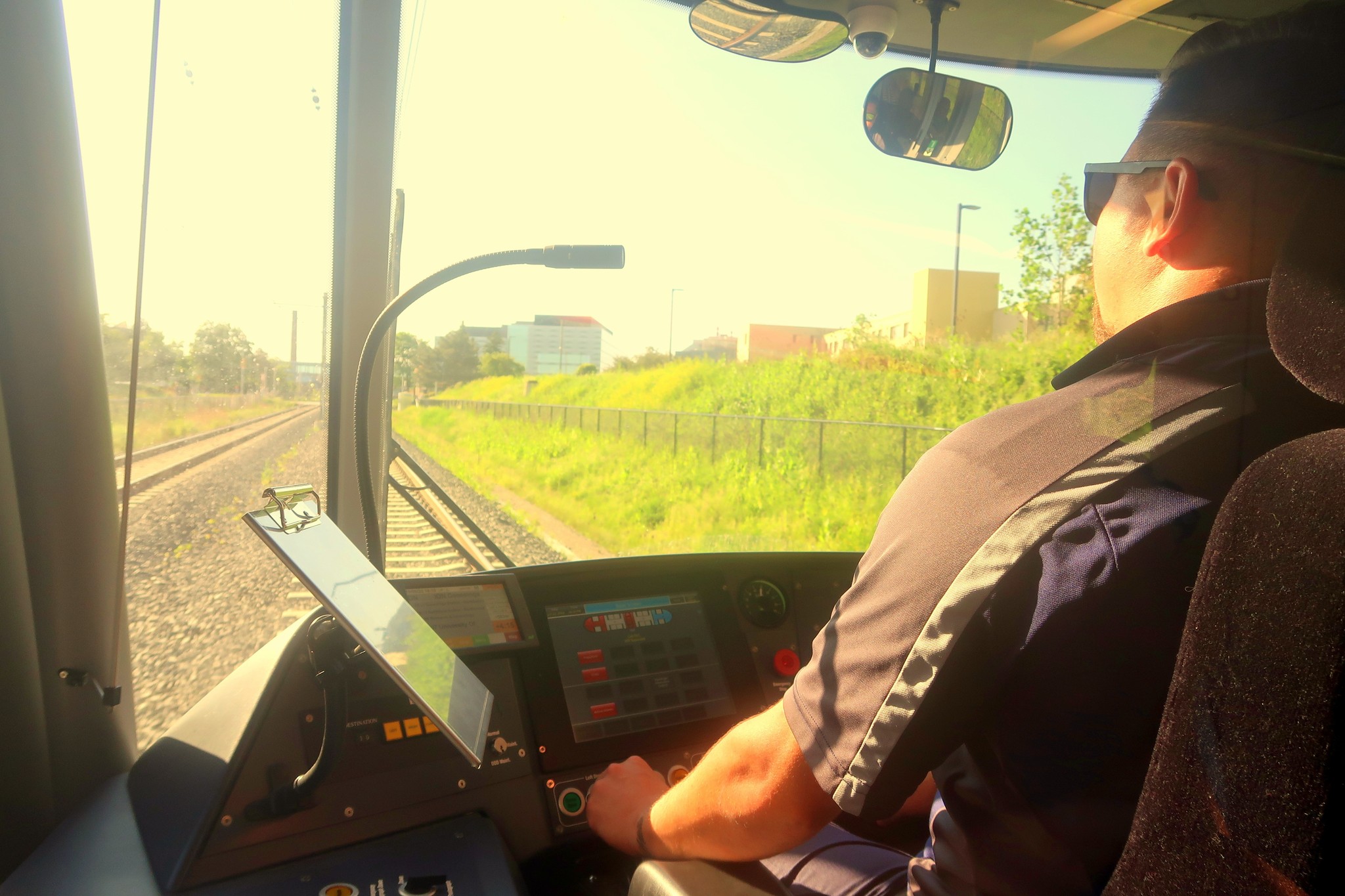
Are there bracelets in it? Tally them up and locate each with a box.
[636,816,661,860]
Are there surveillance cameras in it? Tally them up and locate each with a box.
[846,5,897,60]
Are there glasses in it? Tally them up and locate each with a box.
[1081,159,1217,224]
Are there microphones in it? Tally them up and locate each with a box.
[352,245,624,585]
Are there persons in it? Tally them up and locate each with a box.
[866,95,886,151]
[585,13,1345,896]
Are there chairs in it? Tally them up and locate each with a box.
[1104,263,1345,896]
